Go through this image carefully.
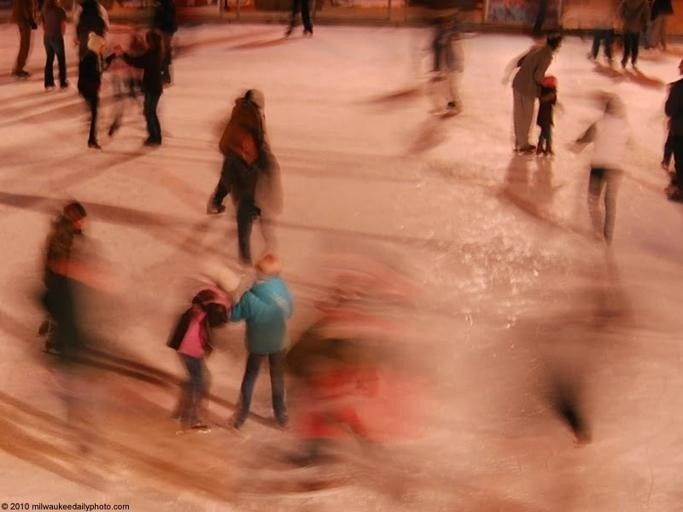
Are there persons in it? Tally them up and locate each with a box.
[588,2,669,70]
[33,202,91,366]
[204,89,270,215]
[427,24,464,113]
[286,1,312,35]
[12,2,175,154]
[282,267,384,475]
[535,74,555,150]
[227,252,294,429]
[660,63,681,201]
[164,283,238,435]
[508,35,562,153]
[221,125,280,263]
[572,96,629,240]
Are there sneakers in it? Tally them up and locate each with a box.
[207,204,227,214]
[516,144,555,158]
[11,65,171,149]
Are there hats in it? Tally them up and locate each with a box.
[246,89,265,108]
[543,77,555,88]
[257,256,281,278]
[216,268,239,294]
